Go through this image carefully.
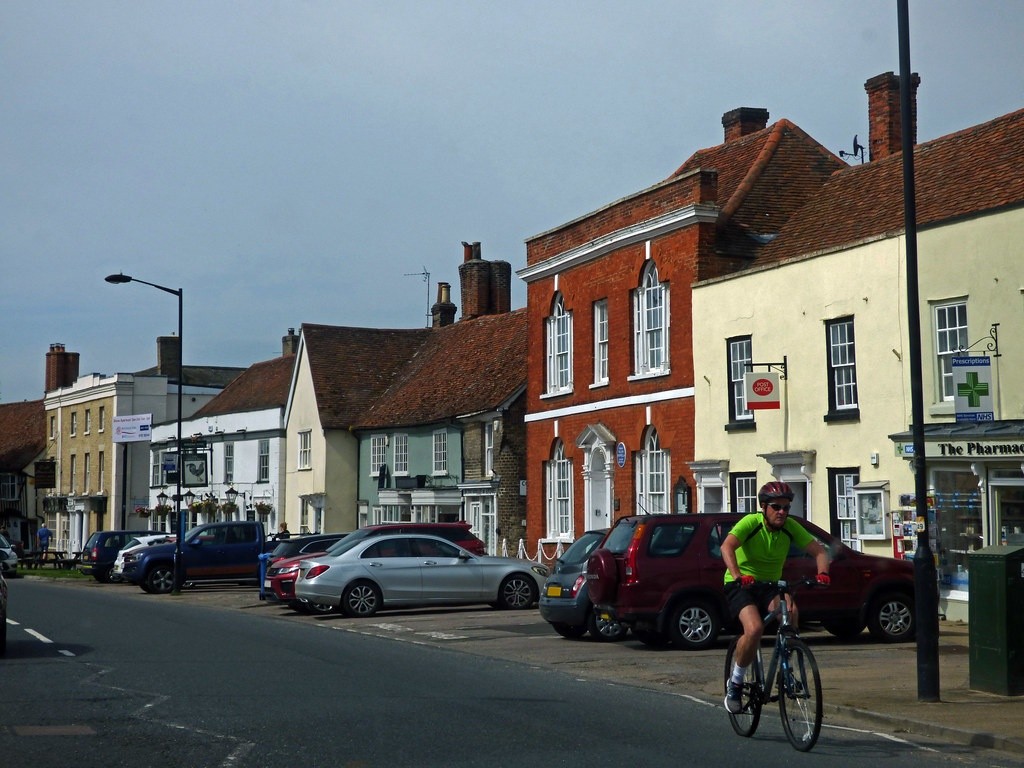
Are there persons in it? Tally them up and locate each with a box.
[722,481,831,714]
[0,525,10,544]
[37,523,53,565]
[279,522,290,539]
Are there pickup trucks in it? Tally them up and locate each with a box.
[122,521,296,593]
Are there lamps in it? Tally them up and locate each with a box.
[156,489,176,506]
[223,486,246,503]
[183,488,202,506]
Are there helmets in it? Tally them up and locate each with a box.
[759,481,795,508]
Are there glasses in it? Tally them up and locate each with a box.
[766,502,792,512]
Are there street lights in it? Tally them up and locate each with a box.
[103,273,186,587]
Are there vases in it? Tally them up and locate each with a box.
[139,512,148,518]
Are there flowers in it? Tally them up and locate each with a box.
[188,502,203,513]
[134,505,149,513]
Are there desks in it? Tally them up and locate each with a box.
[33,551,68,569]
[73,552,83,559]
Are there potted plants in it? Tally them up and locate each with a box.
[202,500,273,515]
[155,505,172,516]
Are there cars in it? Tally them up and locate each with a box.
[587,511,916,646]
[264,518,485,616]
[541,533,633,638]
[115,533,177,585]
[293,534,554,615]
[80,531,160,584]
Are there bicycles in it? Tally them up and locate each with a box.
[723,575,832,751]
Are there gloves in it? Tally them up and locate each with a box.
[816,572,830,587]
[736,575,754,591]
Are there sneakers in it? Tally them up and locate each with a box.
[725,677,743,714]
[775,672,802,693]
